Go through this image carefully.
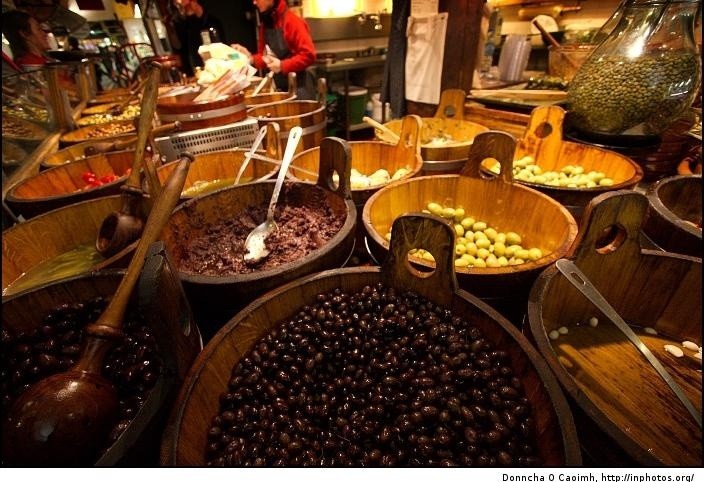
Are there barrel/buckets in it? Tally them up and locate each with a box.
[0,72,703,467]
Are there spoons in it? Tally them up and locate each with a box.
[243,124,303,264]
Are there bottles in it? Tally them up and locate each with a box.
[365,101,372,117]
[565,2,701,139]
[198,31,211,66]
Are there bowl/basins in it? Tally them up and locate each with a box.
[497,35,530,83]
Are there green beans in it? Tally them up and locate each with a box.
[569,52,700,133]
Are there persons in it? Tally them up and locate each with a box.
[67,34,82,51]
[2,10,78,98]
[231,0,316,92]
[162,0,225,76]
